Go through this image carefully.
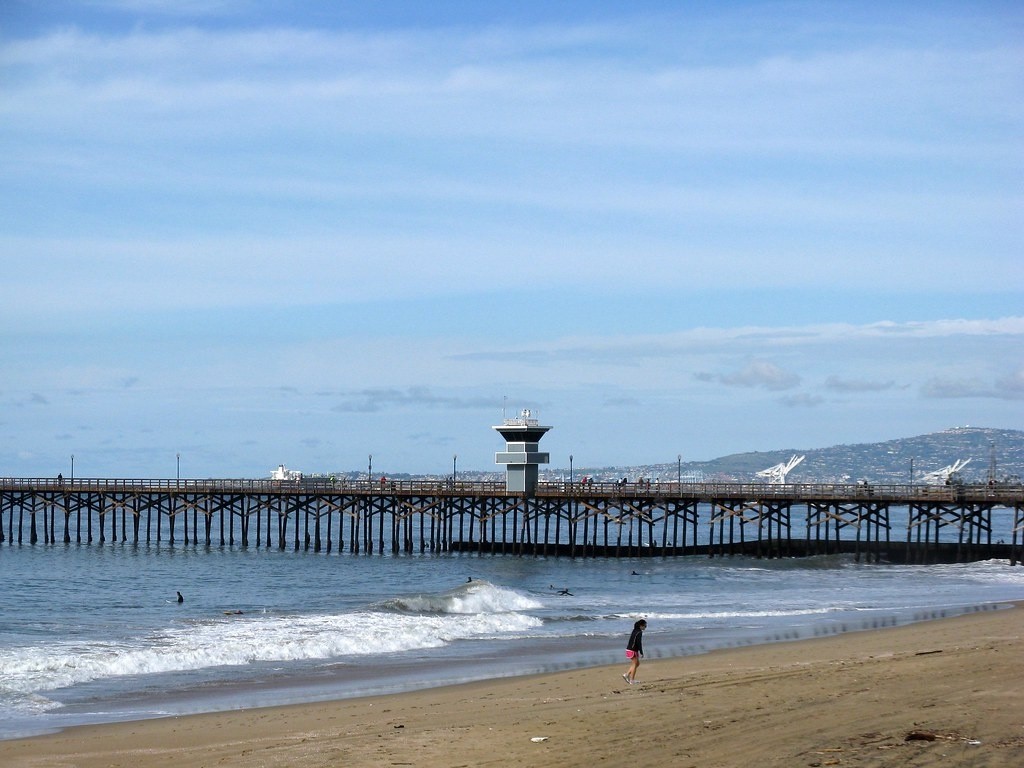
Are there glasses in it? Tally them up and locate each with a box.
[643,624,647,628]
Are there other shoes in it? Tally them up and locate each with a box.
[629,681,640,685]
[623,674,630,684]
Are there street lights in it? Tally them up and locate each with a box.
[71,454,75,490]
[368,454,372,491]
[453,453,457,491]
[678,454,681,493]
[176,453,180,491]
[569,454,574,492]
[910,458,914,494]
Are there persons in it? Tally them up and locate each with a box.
[447,477,454,491]
[176,592,183,603]
[645,479,651,496]
[655,478,660,493]
[589,477,593,491]
[639,477,644,493]
[618,478,622,492]
[581,476,587,490]
[946,474,953,496]
[58,473,62,485]
[622,620,647,685]
[856,480,874,496]
[380,476,387,491]
[988,475,997,496]
